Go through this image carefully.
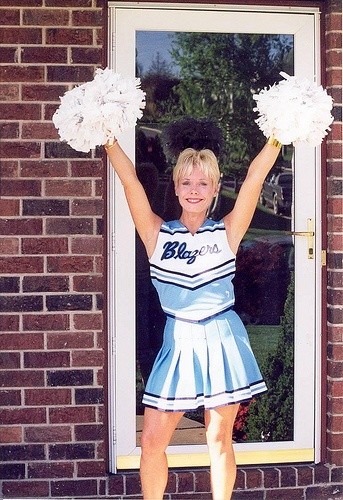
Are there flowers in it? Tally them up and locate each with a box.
[52,66,146,154]
[252,70,335,146]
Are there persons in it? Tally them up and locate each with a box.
[52,68,334,500]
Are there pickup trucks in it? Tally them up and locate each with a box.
[260,170,292,215]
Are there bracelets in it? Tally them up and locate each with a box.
[105,140,119,150]
[268,138,281,148]
